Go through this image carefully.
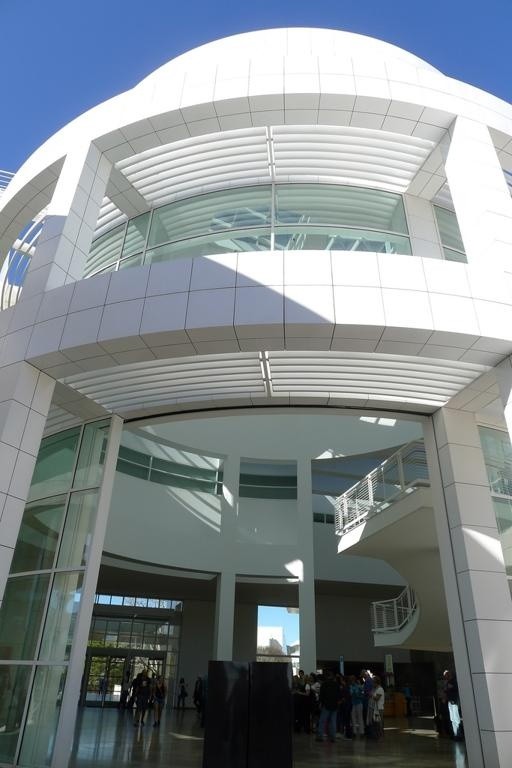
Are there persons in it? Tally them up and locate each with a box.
[120,669,465,744]
